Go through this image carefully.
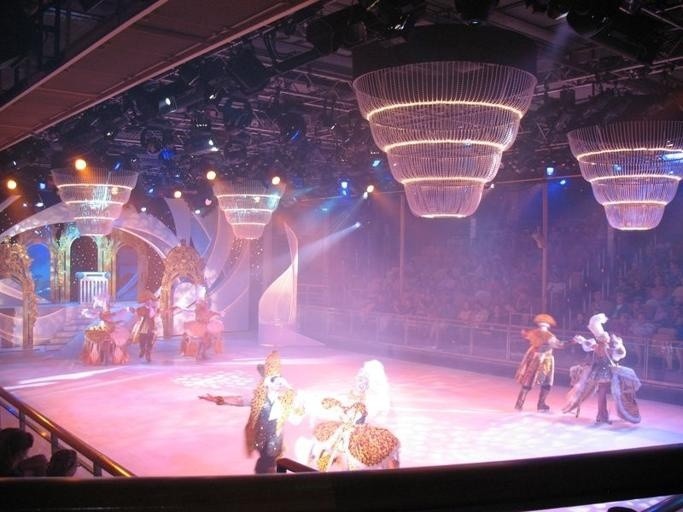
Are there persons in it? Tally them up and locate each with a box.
[0,427,34,472]
[79,292,135,366]
[46,449,78,476]
[127,290,177,362]
[513,312,577,413]
[198,349,308,473]
[308,358,388,472]
[177,297,226,360]
[360,223,683,372]
[561,312,641,425]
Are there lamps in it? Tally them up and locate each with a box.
[563,111,682,233]
[49,43,338,240]
[348,52,540,226]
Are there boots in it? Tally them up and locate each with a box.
[515,389,528,408]
[140,347,151,362]
[537,387,549,409]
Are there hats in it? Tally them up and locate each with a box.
[589,320,606,339]
[533,314,556,326]
